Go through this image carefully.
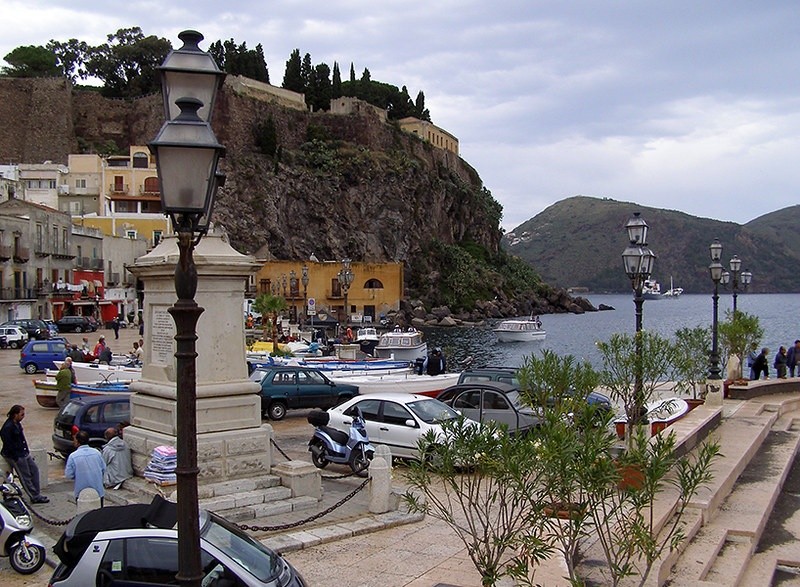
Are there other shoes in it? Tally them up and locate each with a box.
[781,375,786,379]
[764,376,770,379]
[31,496,49,503]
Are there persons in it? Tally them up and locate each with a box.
[63,335,112,366]
[335,336,342,343]
[347,324,354,343]
[750,347,770,380]
[101,428,134,487]
[0,405,50,503]
[65,431,106,512]
[310,339,319,354]
[113,318,120,340]
[422,346,446,375]
[785,340,800,378]
[747,342,758,380]
[55,357,78,410]
[774,347,788,379]
[129,340,144,365]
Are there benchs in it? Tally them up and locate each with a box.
[728,377,800,401]
[645,404,722,466]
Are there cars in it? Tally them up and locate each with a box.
[433,381,575,452]
[41,319,83,351]
[51,494,306,587]
[18,340,83,374]
[324,394,508,471]
[52,316,92,333]
[85,316,100,331]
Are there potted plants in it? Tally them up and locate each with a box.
[514,308,764,518]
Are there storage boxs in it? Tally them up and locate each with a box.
[307,411,330,427]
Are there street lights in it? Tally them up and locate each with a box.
[620,212,663,445]
[707,237,725,395]
[719,254,752,353]
[149,29,228,586]
[301,264,311,323]
[336,255,356,329]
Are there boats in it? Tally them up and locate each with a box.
[32,360,143,408]
[631,274,684,301]
[248,311,548,403]
[613,396,690,440]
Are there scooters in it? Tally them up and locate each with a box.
[0,468,47,576]
[307,405,376,478]
[0,336,8,349]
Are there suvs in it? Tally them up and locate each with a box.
[0,324,29,350]
[249,365,360,421]
[1,319,51,342]
[457,365,613,425]
[50,394,132,458]
